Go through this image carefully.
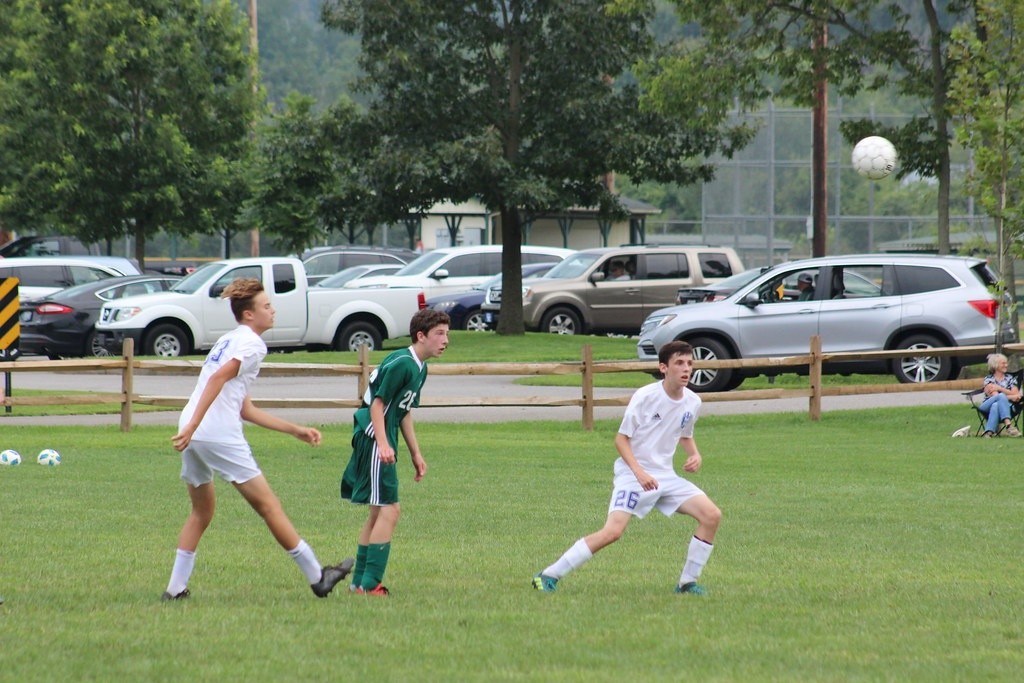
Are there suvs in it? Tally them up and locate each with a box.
[0,255,144,301]
[635,253,1020,393]
[481,244,748,336]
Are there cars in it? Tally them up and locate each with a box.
[676,265,882,304]
[426,262,557,333]
[283,245,579,300]
[18,275,183,359]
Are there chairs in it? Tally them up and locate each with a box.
[961,369,1024,438]
[625,261,636,273]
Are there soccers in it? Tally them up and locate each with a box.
[38,449,61,466]
[0,450,21,466]
[852,135,898,179]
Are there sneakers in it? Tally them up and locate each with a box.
[161,588,190,602]
[310,558,354,598]
[1006,426,1022,438]
[674,582,704,595]
[980,432,994,438]
[357,584,389,594]
[532,572,559,593]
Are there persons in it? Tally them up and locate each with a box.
[607,260,631,281]
[979,354,1022,438]
[161,279,354,602]
[798,274,814,301]
[341,309,450,596]
[532,341,721,593]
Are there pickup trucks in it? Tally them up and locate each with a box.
[0,234,199,276]
[94,258,427,356]
